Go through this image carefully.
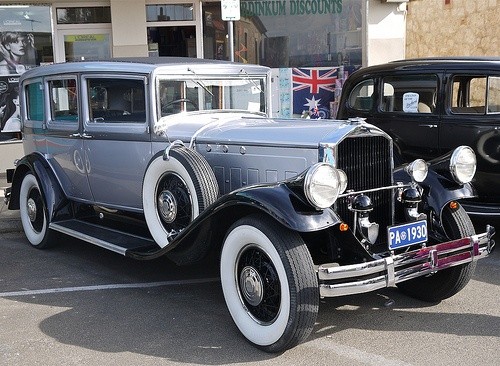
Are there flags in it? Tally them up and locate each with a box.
[292,67,341,114]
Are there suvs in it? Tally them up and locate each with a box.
[337,57,500,242]
[3,57,496,352]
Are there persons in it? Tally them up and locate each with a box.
[0,31,35,73]
[0,84,22,132]
